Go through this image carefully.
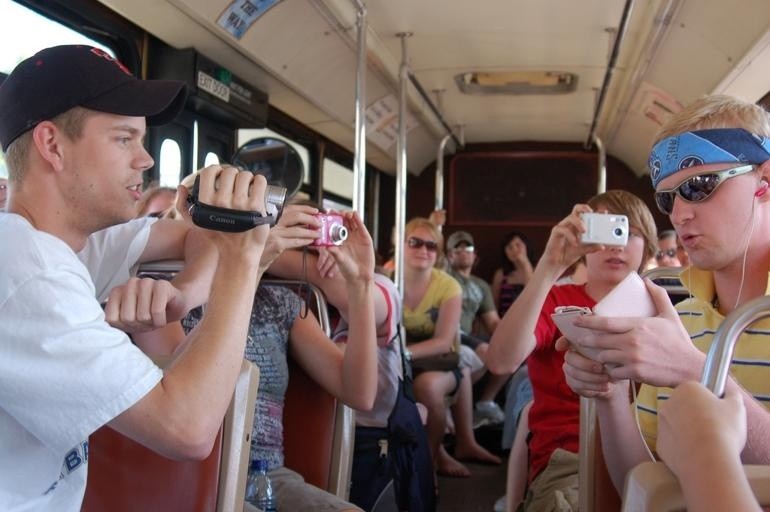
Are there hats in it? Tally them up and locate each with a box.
[447,230,474,248]
[0,43,192,155]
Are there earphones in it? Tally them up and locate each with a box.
[755,180,770,196]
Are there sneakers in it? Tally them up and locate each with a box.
[464,400,504,430]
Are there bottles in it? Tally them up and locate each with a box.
[246,458,274,511]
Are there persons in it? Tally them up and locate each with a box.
[550,91,770,500]
[482,189,663,510]
[134,183,693,482]
[1,43,276,512]
[652,378,766,512]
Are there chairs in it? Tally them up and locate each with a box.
[233,272,358,504]
[81,259,262,512]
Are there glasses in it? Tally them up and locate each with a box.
[655,248,676,260]
[405,236,475,254]
[653,162,756,214]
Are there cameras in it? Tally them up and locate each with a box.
[186,175,288,228]
[307,214,348,248]
[577,212,629,247]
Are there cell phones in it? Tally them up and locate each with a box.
[552,271,659,382]
